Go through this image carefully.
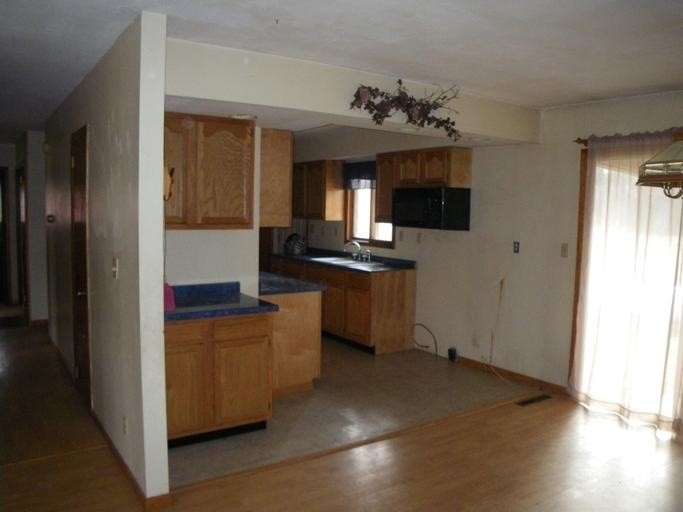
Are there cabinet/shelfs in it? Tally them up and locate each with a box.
[162,112,255,230]
[167,283,279,441]
[259,272,326,399]
[374,146,469,224]
[261,128,293,229]
[273,245,418,356]
[294,159,345,222]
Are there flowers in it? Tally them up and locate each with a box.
[348,84,464,143]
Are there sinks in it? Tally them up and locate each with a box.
[333,260,389,267]
[310,256,357,263]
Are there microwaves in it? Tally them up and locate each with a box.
[392,186,468,231]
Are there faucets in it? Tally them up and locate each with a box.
[343,242,361,260]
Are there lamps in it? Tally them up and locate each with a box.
[636,126,683,199]
